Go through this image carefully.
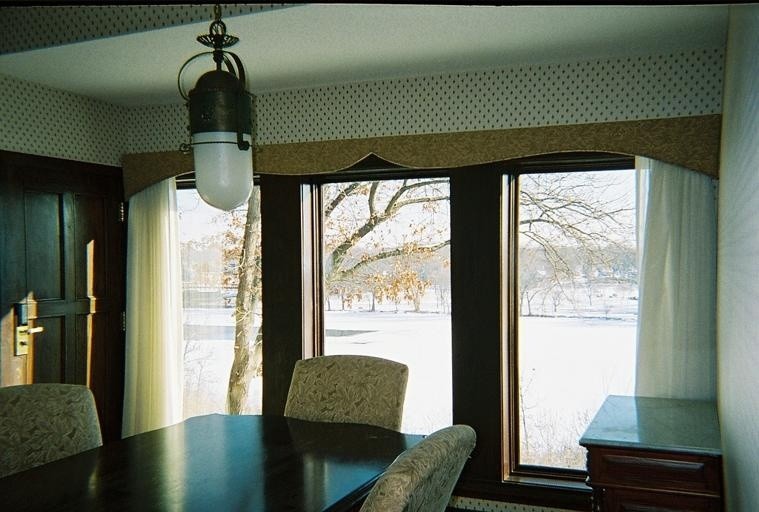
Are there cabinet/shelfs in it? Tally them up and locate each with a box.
[579,395,726,512]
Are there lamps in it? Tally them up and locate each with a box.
[177,3,254,212]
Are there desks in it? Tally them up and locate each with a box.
[0,413,429,512]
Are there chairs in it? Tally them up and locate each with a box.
[359,424,476,512]
[0,383,104,480]
[284,355,409,432]
